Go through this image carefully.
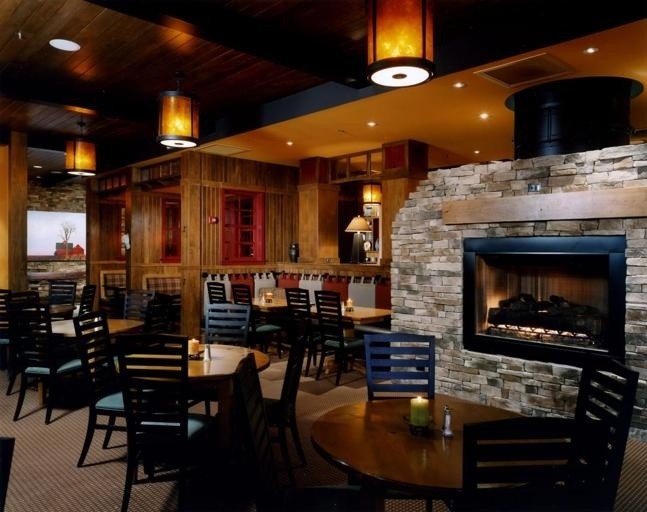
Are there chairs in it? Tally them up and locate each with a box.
[1,279,645,512]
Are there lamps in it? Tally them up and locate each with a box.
[156,71,201,148]
[344,215,371,264]
[365,1,435,88]
[62,113,97,176]
[361,182,382,206]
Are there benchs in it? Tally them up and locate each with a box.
[99,269,125,318]
[143,273,181,306]
